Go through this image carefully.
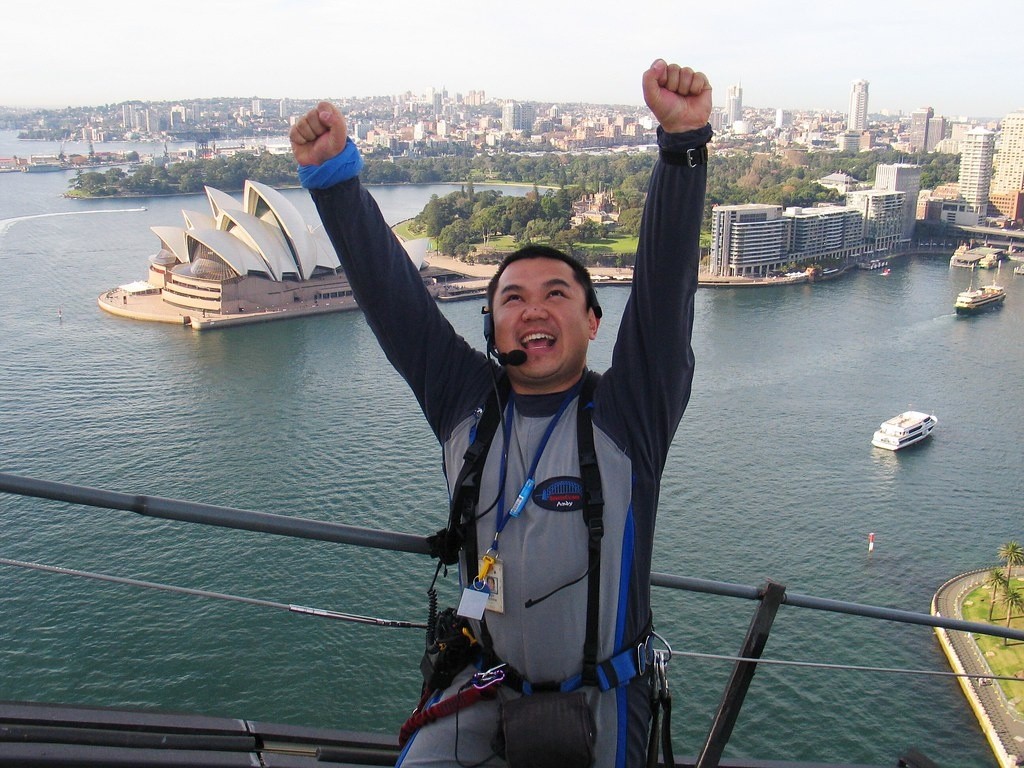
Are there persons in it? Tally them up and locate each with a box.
[487,577,497,594]
[289,58,714,768]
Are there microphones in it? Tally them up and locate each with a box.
[484,311,526,367]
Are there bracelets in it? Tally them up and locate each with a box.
[658,146,709,168]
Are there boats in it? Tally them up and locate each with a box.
[978,254,997,269]
[953,280,1007,316]
[871,411,938,451]
[438,290,487,302]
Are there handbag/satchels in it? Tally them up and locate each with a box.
[500,692,598,768]
